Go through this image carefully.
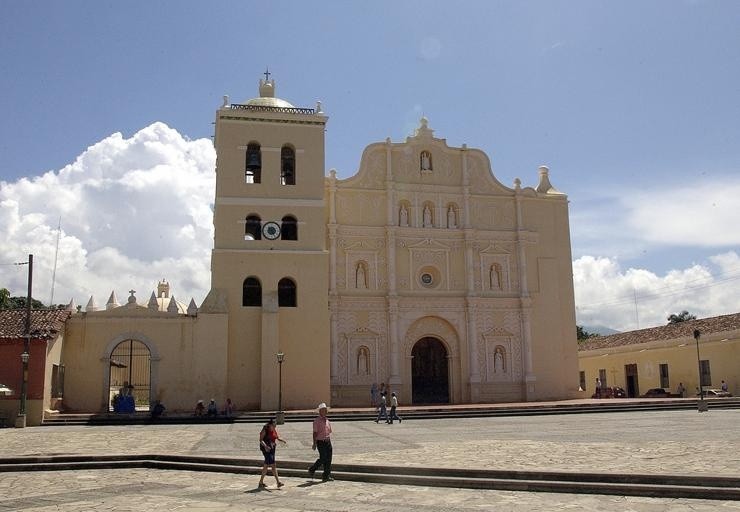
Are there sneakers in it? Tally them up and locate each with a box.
[259,482,284,489]
[375,418,402,424]
[307,467,335,482]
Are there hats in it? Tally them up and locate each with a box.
[317,402,330,410]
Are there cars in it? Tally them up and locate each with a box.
[697,388,732,397]
[1,384,16,399]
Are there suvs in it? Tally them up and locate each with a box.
[592,386,626,398]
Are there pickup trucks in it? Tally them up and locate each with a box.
[639,389,683,398]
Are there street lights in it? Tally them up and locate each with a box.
[277,353,286,412]
[693,329,704,400]
[19,352,30,415]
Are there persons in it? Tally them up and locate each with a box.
[308,402,334,482]
[696,387,701,398]
[424,205,433,227]
[495,347,504,371]
[380,383,386,397]
[258,418,285,487]
[358,349,367,374]
[371,383,378,407]
[151,400,165,417]
[357,263,366,289]
[593,378,602,399]
[490,265,500,289]
[721,380,727,391]
[224,398,233,416]
[447,205,455,230]
[400,203,407,227]
[208,399,218,417]
[677,382,685,397]
[388,392,402,424]
[194,400,205,418]
[421,151,431,170]
[374,393,390,423]
